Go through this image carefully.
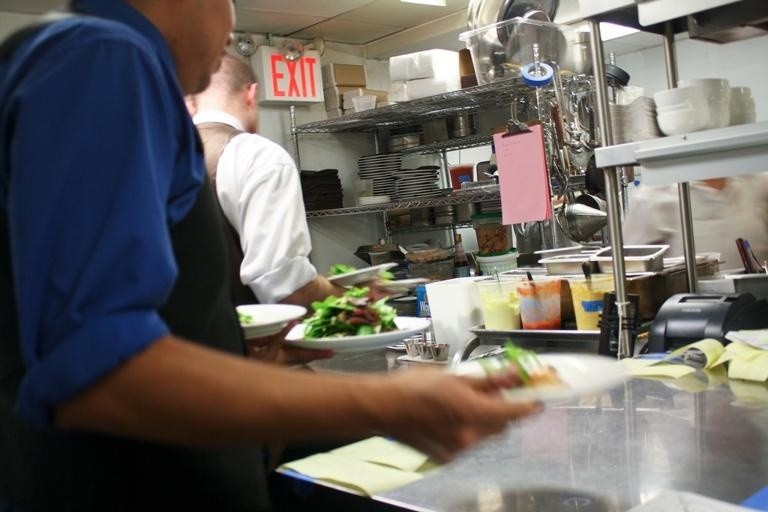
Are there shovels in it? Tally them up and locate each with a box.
[550,132,567,189]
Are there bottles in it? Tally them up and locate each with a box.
[451,233,470,278]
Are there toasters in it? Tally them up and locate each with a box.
[647,290,766,356]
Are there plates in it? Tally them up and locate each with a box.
[607,97,659,145]
[287,312,434,354]
[392,167,452,199]
[324,261,398,288]
[438,347,627,404]
[358,193,391,207]
[380,275,429,293]
[235,301,309,342]
[357,151,401,196]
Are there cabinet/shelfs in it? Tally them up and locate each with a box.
[282,48,625,283]
[586,3,767,362]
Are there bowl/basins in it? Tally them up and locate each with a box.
[654,77,731,135]
[729,86,758,124]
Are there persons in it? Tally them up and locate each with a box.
[177,53,398,356]
[4,0,545,512]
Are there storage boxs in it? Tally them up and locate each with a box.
[321,62,389,118]
[457,16,596,89]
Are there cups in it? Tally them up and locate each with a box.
[351,178,373,206]
[476,273,615,331]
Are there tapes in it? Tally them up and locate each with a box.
[521,62,554,86]
